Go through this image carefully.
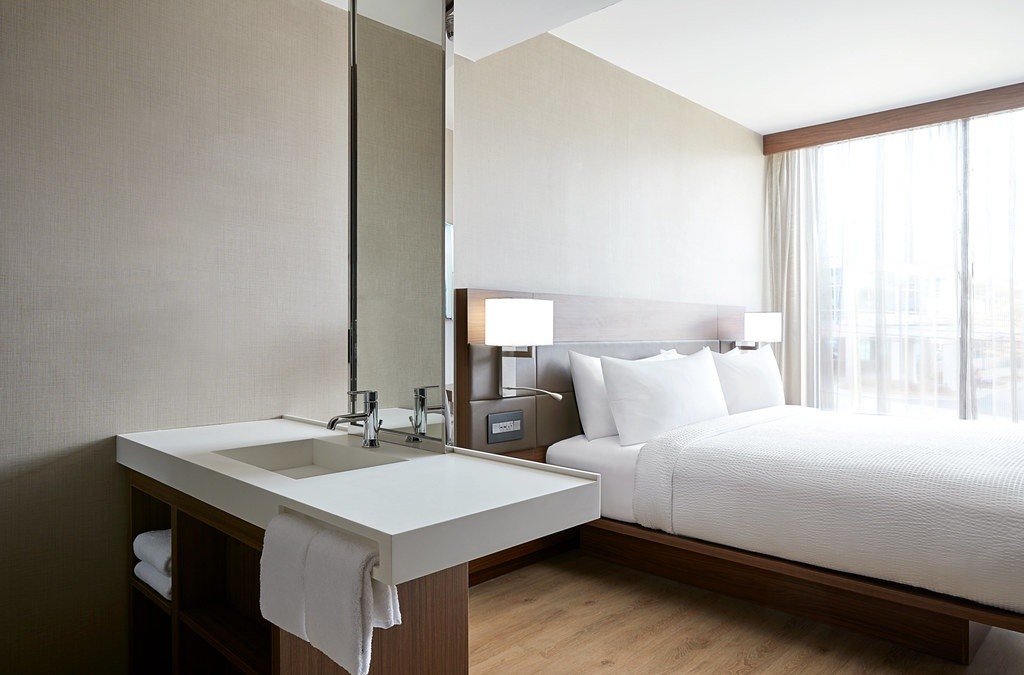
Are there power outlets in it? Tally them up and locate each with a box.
[487,410,524,444]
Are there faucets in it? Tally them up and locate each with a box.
[408,384,445,436]
[326,389,381,449]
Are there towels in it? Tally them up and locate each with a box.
[257,512,405,675]
[131,526,173,602]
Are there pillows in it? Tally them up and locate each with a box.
[702,343,785,416]
[568,347,678,440]
[660,347,742,357]
[599,346,729,445]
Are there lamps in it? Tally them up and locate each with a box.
[717,310,782,351]
[509,386,562,403]
[483,298,554,397]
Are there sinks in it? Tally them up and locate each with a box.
[211,437,411,481]
[389,421,444,440]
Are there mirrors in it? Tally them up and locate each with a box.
[348,0,455,447]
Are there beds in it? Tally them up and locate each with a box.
[546,404,1024,664]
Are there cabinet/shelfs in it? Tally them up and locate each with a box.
[129,471,469,675]
